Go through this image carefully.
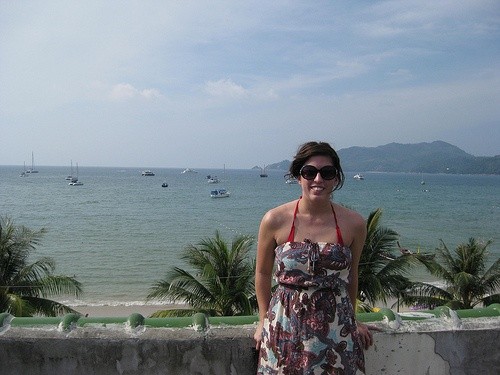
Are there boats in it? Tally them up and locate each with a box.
[141,169,156,176]
[204,175,219,184]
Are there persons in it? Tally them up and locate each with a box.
[254,141,378,375]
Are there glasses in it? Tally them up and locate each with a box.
[299,165,339,180]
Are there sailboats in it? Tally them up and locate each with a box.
[66,159,84,185]
[259,164,269,177]
[353,172,367,180]
[209,164,232,197]
[19,151,40,177]
